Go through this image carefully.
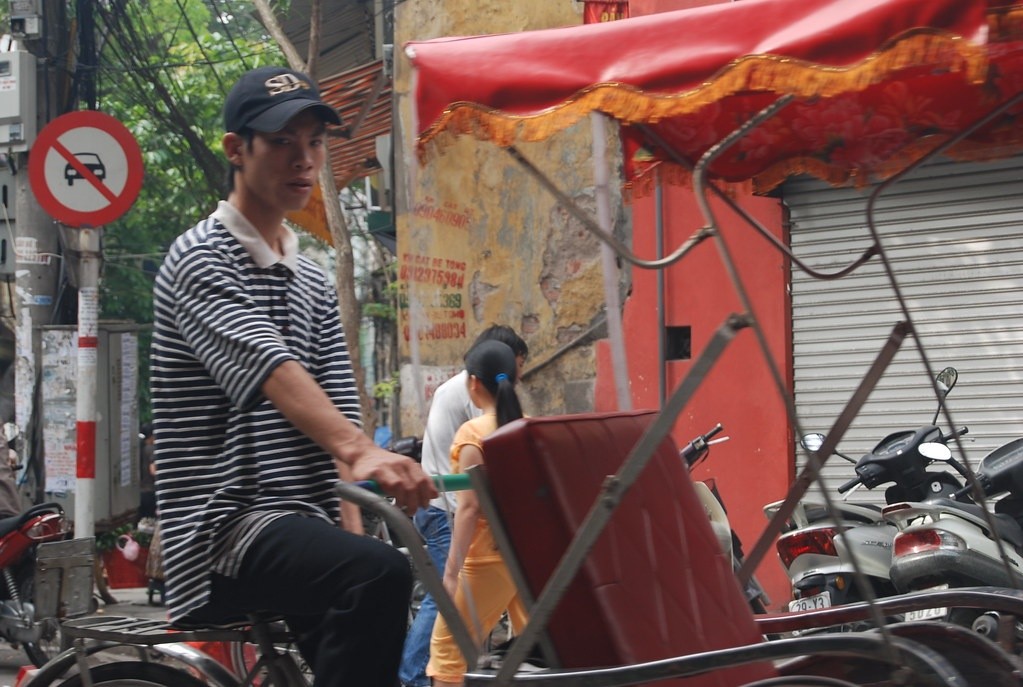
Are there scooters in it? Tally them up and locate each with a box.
[762,366,969,603]
[665,421,766,618]
[882,437,1020,649]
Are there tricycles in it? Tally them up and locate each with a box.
[23,0,1022,687]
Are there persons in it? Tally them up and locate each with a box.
[427,340,533,686]
[153,67,439,687]
[141,425,155,475]
[400,325,529,685]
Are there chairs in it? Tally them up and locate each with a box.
[330,404,1023,687]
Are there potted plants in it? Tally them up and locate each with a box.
[96,521,154,590]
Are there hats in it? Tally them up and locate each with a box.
[226,66,344,135]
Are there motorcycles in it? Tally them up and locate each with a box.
[0,419,101,667]
